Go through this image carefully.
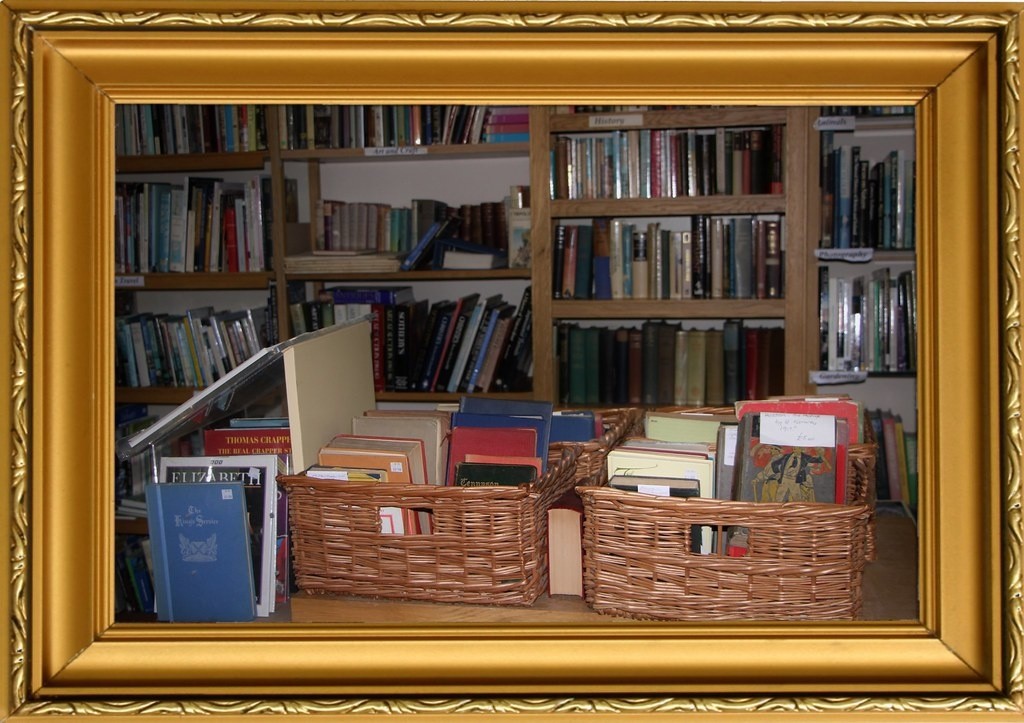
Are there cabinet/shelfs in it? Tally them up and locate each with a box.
[114,103,918,625]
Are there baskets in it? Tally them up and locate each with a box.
[573,460,871,622]
[275,442,577,607]
[624,406,878,564]
[544,407,636,487]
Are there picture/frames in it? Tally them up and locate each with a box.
[0,0,1024,723]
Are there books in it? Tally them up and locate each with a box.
[145,482,257,623]
[116,104,919,622]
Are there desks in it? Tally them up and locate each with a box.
[290,500,920,623]
